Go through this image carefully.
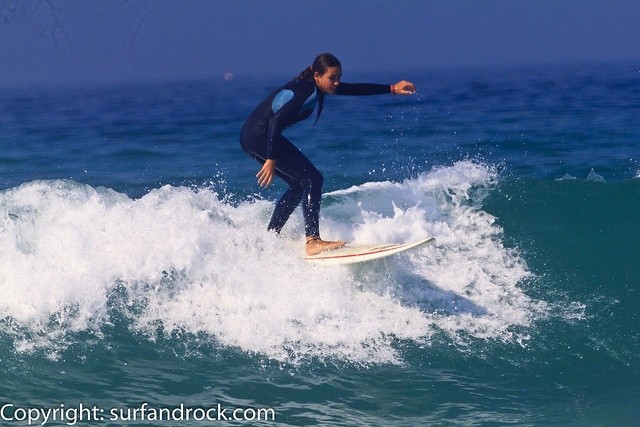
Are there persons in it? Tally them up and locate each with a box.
[239,52,415,255]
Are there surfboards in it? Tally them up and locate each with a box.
[303,236,436,266]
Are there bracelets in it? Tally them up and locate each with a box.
[392,85,395,93]
